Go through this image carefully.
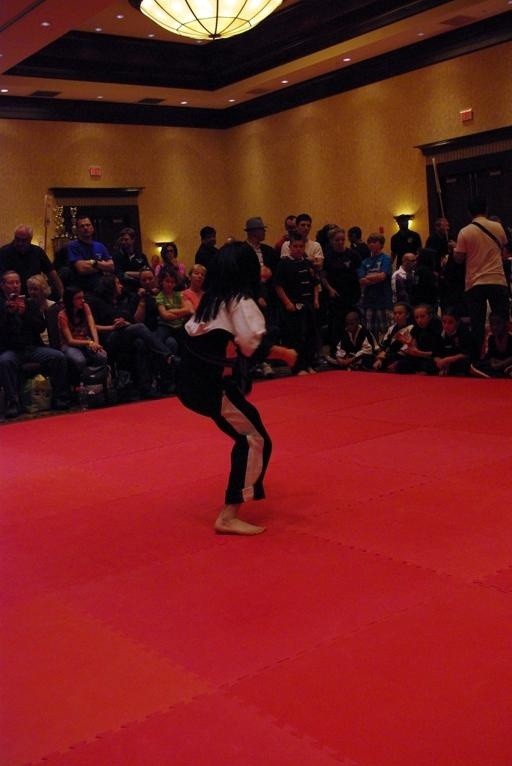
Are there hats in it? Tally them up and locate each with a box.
[396,214,410,222]
[243,217,268,232]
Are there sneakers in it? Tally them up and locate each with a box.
[255,361,276,378]
[467,363,490,378]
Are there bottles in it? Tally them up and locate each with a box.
[79,381,89,408]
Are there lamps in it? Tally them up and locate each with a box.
[128,0,290,43]
[390,211,415,224]
[155,240,173,249]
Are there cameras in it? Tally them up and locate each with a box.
[7,291,26,303]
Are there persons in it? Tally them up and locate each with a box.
[243,197,512,382]
[176,241,297,535]
[1,206,218,416]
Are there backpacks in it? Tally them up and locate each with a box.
[79,363,116,409]
